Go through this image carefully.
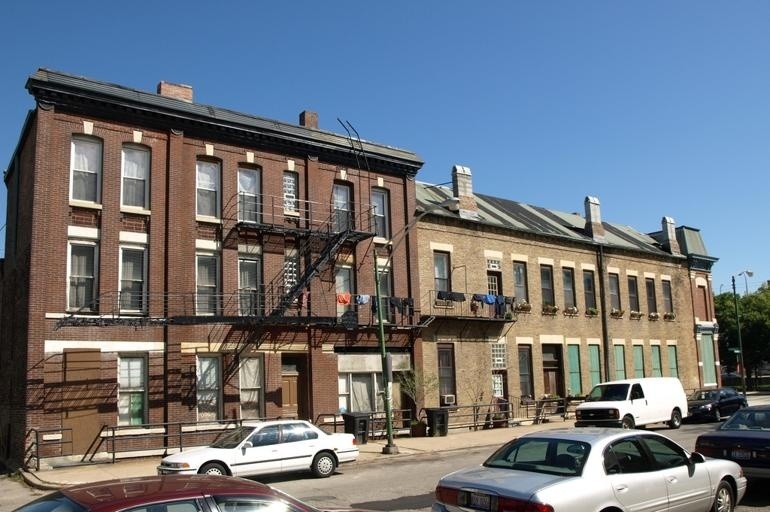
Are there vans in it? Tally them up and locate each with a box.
[572,377,689,437]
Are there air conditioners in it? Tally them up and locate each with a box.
[439,393,458,406]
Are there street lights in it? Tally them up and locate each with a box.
[730,269,757,402]
[719,283,724,295]
[370,195,464,456]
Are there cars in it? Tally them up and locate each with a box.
[151,418,363,481]
[720,370,742,386]
[690,403,770,482]
[685,387,750,425]
[428,426,753,512]
[5,471,384,512]
